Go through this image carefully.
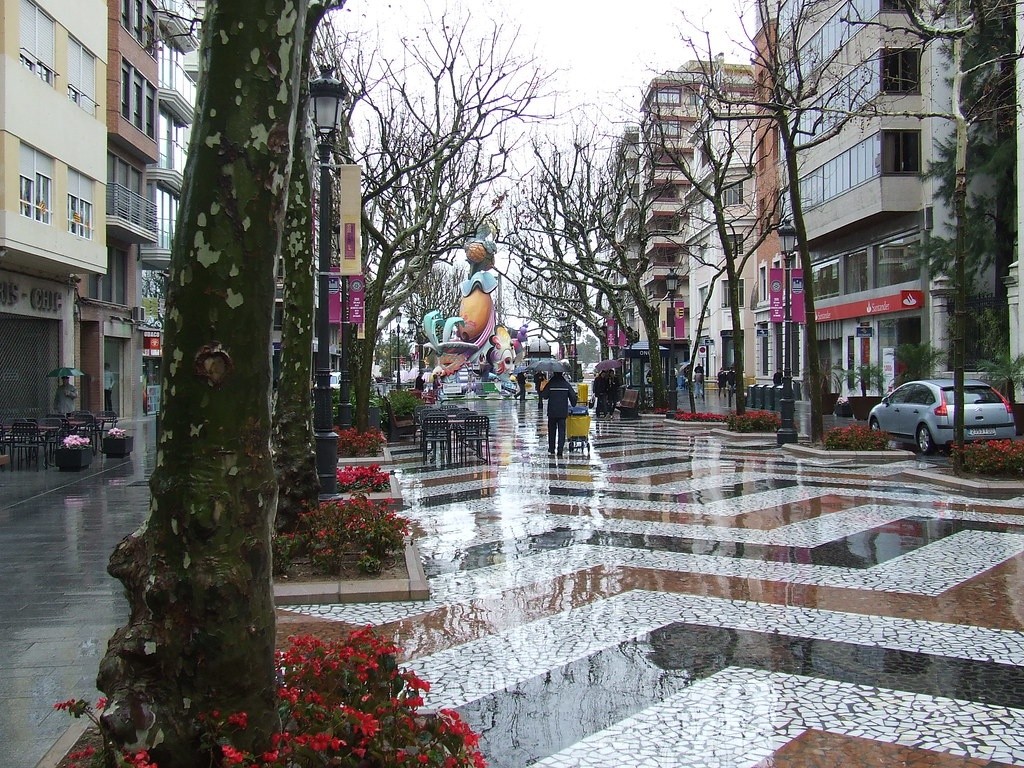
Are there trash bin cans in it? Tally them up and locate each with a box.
[775,385,784,411]
[746,384,758,409]
[764,385,776,411]
[754,384,768,409]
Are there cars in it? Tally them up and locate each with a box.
[868,378,1017,456]
[313,372,341,390]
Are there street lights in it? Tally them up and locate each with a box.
[571,314,578,383]
[665,267,680,419]
[307,64,349,506]
[776,220,799,445]
[395,309,402,391]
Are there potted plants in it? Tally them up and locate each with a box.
[800,360,887,420]
[976,352,1024,435]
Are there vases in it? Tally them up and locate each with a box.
[54,449,94,472]
[103,436,134,458]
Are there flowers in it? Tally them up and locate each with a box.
[61,435,93,448]
[108,427,129,439]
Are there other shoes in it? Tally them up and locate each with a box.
[548,448,555,454]
[514,395,518,400]
[604,414,614,421]
[521,399,526,402]
[538,403,543,409]
[557,451,562,457]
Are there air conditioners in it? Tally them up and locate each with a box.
[132,306,146,322]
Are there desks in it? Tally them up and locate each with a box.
[0,426,59,469]
[96,417,111,434]
[448,420,484,456]
[63,422,86,434]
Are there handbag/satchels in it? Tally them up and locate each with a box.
[540,377,549,391]
[589,393,595,408]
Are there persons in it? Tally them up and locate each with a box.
[716,367,727,396]
[415,372,425,392]
[53,376,77,422]
[593,369,620,419]
[727,367,735,396]
[537,371,578,456]
[773,368,783,385]
[683,367,690,390]
[534,371,547,407]
[514,372,528,401]
[694,363,704,398]
[433,374,442,400]
[646,370,653,397]
[104,362,115,412]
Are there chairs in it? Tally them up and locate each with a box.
[0,411,117,472]
[387,399,491,465]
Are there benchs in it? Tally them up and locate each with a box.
[613,389,642,418]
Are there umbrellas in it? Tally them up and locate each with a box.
[417,368,432,372]
[513,366,529,374]
[525,361,574,372]
[46,367,85,377]
[679,363,690,372]
[595,360,622,371]
